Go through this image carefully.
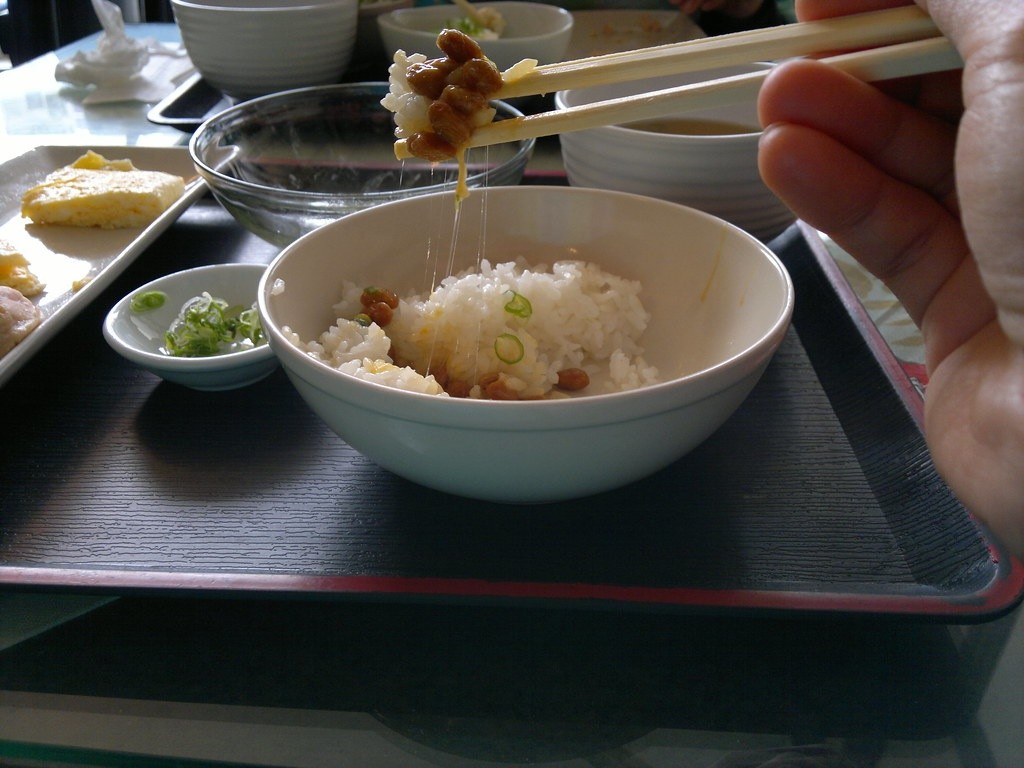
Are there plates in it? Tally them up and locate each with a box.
[0,145,243,378]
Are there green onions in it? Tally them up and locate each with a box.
[128,289,270,357]
[494,289,533,364]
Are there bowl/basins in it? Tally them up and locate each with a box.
[169,0,359,101]
[103,261,280,393]
[556,61,802,240]
[189,82,538,251]
[378,3,574,73]
[257,183,796,505]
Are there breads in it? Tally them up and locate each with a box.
[0,239,47,358]
[20,150,184,228]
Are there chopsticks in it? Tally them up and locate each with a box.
[452,0,487,26]
[392,5,965,159]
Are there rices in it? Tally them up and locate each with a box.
[285,45,663,399]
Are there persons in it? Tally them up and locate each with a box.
[756,0,1024,558]
